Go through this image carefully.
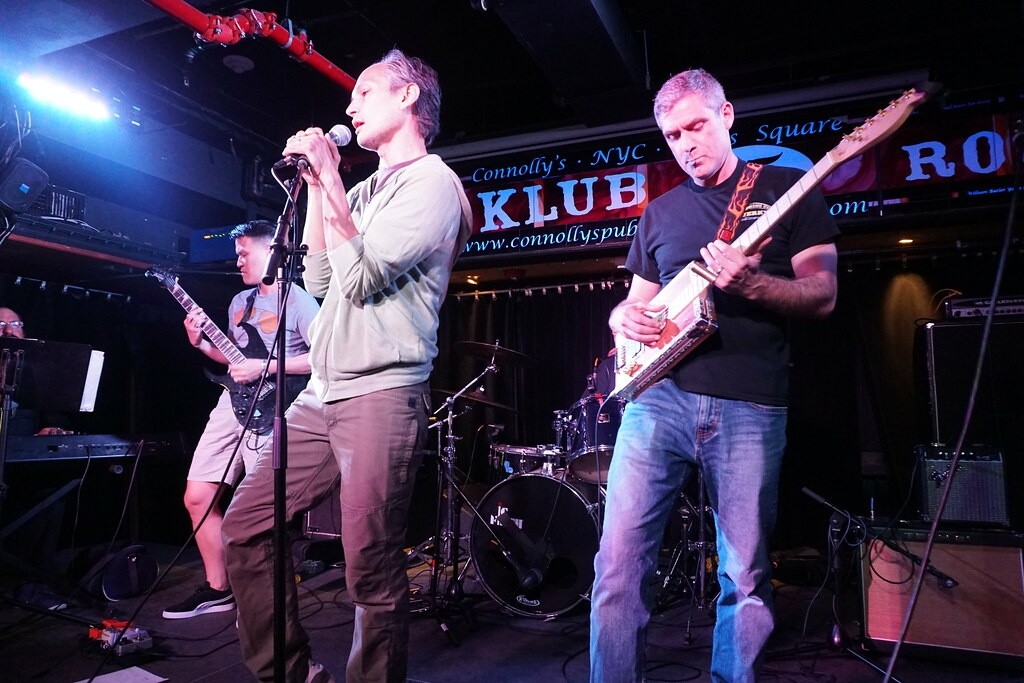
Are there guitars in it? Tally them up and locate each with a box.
[144,263,307,435]
[614,87,927,403]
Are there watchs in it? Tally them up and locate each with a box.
[262,360,270,378]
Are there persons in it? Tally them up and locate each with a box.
[221,51,471,683]
[0,305,67,612]
[163,218,321,618]
[588,68,838,683]
[581,356,615,396]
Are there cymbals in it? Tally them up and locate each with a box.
[452,341,538,371]
[430,386,517,414]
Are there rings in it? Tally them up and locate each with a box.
[196,325,199,328]
[298,139,301,145]
[717,267,722,274]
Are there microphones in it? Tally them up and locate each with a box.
[592,356,600,391]
[481,424,505,431]
[520,568,542,589]
[271,125,352,171]
[944,580,955,589]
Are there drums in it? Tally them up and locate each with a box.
[468,467,607,619]
[565,395,630,486]
[488,443,563,486]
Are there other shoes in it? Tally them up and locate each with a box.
[305,659,335,683]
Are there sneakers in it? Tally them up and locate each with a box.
[163,580,236,618]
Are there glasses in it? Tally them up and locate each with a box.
[0,320,23,329]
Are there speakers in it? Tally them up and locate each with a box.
[854,317,1024,663]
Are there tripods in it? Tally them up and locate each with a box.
[408,354,501,649]
[652,470,720,646]
[760,488,958,683]
